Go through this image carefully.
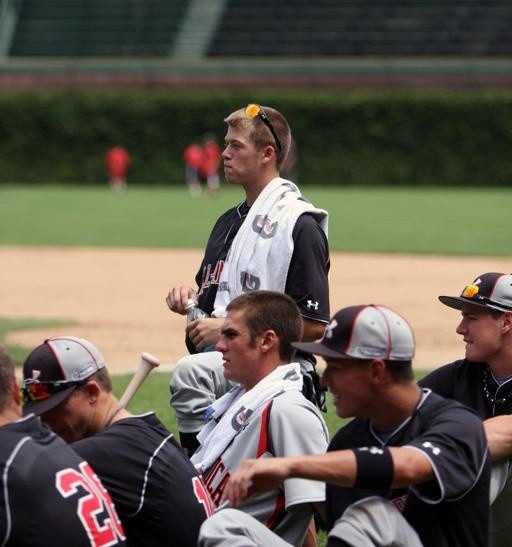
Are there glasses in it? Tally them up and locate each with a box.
[245,103,282,151]
[461,284,512,311]
[19,379,78,403]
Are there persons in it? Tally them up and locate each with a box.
[201,130,225,190]
[0,342,133,545]
[196,300,492,546]
[414,269,511,547]
[180,138,205,196]
[106,143,133,192]
[20,334,218,546]
[182,291,335,546]
[163,100,332,458]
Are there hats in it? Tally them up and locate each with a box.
[23,335,106,418]
[291,303,415,361]
[438,272,512,314]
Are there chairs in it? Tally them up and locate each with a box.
[0,0,509,61]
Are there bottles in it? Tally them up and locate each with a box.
[184,299,217,351]
